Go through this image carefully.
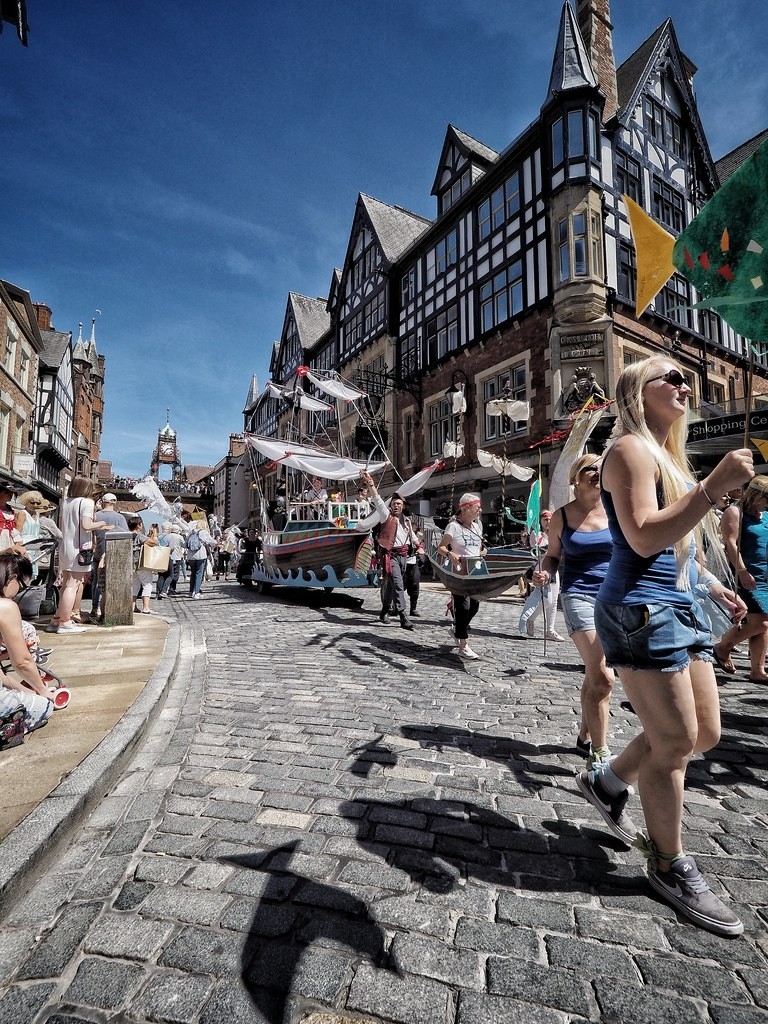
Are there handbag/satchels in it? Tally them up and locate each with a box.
[0,704,26,751]
[78,550,94,565]
[138,539,171,572]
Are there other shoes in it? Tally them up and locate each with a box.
[399,616,413,628]
[576,736,592,753]
[131,577,228,613]
[380,611,391,624]
[410,609,420,616]
[44,609,104,634]
[36,648,52,665]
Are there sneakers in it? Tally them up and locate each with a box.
[448,626,461,647]
[547,631,566,641]
[458,648,479,660]
[648,856,743,935]
[526,619,535,637]
[575,770,640,845]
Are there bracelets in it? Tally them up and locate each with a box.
[547,573,552,585]
[365,477,374,487]
[700,481,714,505]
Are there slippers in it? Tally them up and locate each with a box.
[712,650,736,675]
[748,679,768,685]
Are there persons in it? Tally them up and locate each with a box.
[114,475,212,494]
[526,510,565,642]
[438,493,487,660]
[392,521,423,617]
[516,524,538,598]
[360,469,425,629]
[127,517,263,614]
[34,499,62,554]
[17,491,44,579]
[45,474,115,633]
[701,472,768,684]
[273,477,371,521]
[0,481,22,552]
[533,453,634,794]
[90,492,128,624]
[0,554,54,733]
[575,356,756,942]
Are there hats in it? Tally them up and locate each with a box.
[102,493,118,504]
[0,481,18,499]
[36,499,56,514]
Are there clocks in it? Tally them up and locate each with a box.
[160,443,175,456]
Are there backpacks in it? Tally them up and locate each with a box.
[186,529,203,552]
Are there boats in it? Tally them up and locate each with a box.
[242,364,449,596]
[420,523,545,602]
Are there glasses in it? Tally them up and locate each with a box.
[28,501,41,505]
[16,575,29,594]
[646,370,689,388]
[2,490,13,494]
[582,464,599,471]
